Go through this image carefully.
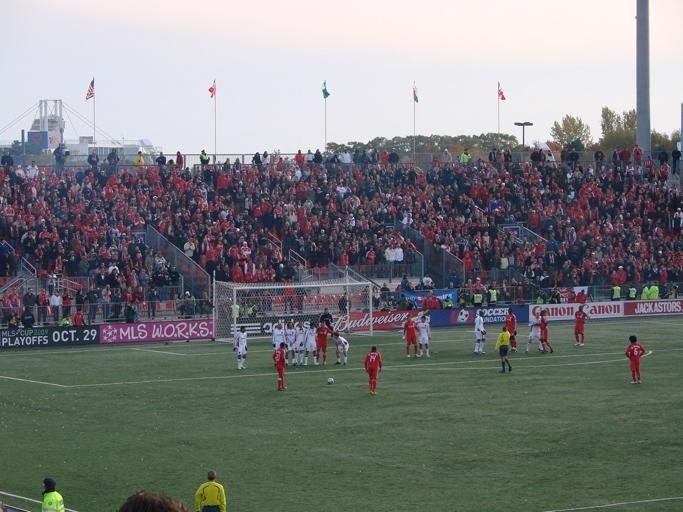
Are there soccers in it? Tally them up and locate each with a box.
[328,378,335,383]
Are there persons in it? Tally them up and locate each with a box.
[416,316,431,358]
[331,332,350,366]
[284,322,295,365]
[364,344,383,396]
[314,320,333,366]
[573,305,591,348]
[1,137,682,327]
[193,469,226,512]
[303,322,319,364]
[114,488,189,512]
[232,325,248,370]
[472,310,487,354]
[531,311,551,353]
[294,320,306,365]
[625,334,645,386]
[271,342,287,392]
[271,323,285,365]
[503,308,518,352]
[402,313,419,358]
[40,478,64,511]
[525,312,544,353]
[494,327,512,374]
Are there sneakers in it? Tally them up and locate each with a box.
[369,391,377,396]
[574,343,584,347]
[630,380,642,384]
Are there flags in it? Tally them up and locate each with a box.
[208,80,216,97]
[85,78,94,102]
[412,85,418,102]
[497,83,506,102]
[321,82,329,99]
